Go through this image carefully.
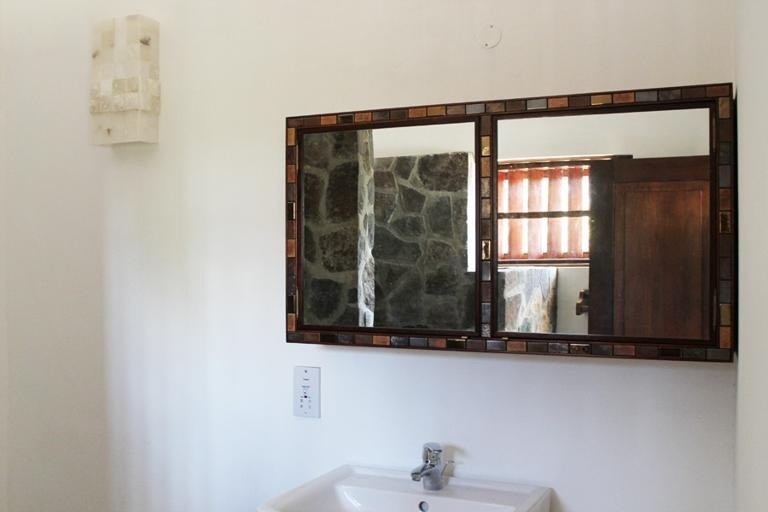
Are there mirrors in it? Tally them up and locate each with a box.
[285,82,737,366]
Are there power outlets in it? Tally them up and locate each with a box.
[293,364,322,419]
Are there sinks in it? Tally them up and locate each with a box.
[256,463,553,512]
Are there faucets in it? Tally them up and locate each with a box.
[411,441,457,491]
